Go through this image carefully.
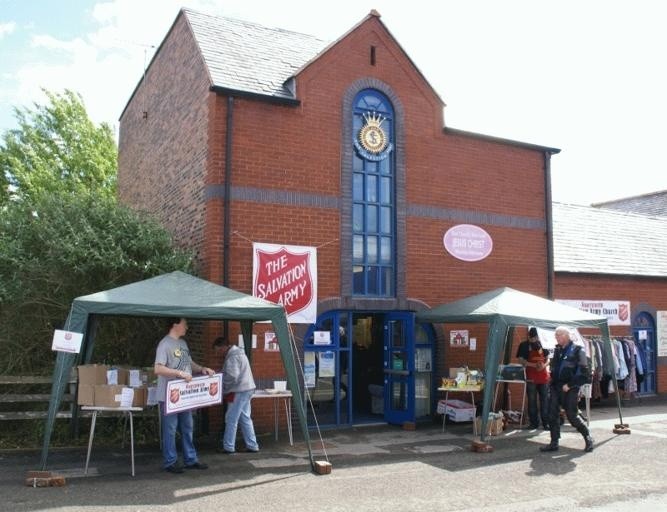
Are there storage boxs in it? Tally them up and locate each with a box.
[77,363,158,409]
[472,417,503,435]
[436,399,478,422]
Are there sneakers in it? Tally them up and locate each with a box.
[213,443,257,453]
[188,462,210,470]
[168,462,183,474]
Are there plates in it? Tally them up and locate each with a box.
[264,388,293,395]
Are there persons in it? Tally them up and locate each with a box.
[540,325,596,454]
[211,337,261,452]
[152,317,215,473]
[516,327,552,430]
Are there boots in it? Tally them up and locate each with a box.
[583,432,594,452]
[540,438,559,452]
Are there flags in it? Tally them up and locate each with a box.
[252,242,318,324]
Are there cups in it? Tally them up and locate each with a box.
[274,380,287,391]
[441,366,478,390]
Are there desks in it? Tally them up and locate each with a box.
[493,380,527,428]
[251,394,293,446]
[81,406,144,477]
[436,385,483,437]
